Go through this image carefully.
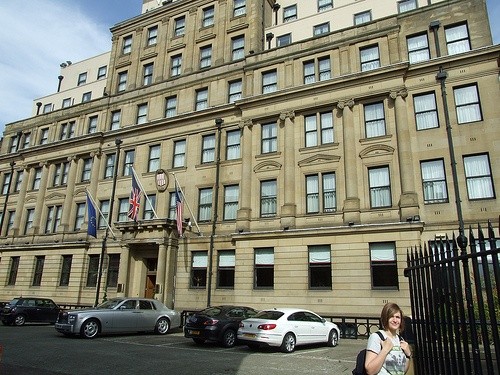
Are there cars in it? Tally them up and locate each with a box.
[236,307,340,353]
[0,296,61,326]
[54,297,181,339]
[183,305,262,348]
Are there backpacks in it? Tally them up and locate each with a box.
[352,331,403,375]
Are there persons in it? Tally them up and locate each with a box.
[365,302,411,375]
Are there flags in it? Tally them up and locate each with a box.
[127,171,142,223]
[86,194,97,239]
[175,181,183,237]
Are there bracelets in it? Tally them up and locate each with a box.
[406,356,410,359]
